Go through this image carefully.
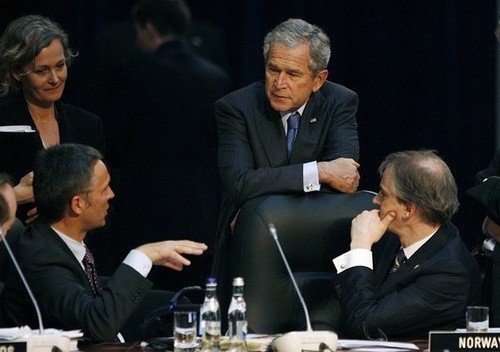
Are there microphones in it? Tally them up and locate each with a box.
[269,223,339,352]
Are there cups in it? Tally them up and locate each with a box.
[171,311,198,352]
[198,311,221,352]
[226,313,248,352]
[465,307,489,331]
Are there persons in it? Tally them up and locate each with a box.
[121,0,235,305]
[212,17,361,336]
[0,144,208,343]
[330,152,484,339]
[0,14,107,227]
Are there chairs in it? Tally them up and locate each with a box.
[228,187,379,335]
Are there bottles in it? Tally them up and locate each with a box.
[199,276,220,337]
[227,277,247,340]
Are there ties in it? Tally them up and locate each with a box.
[382,248,408,285]
[83,244,104,295]
[286,112,301,161]
